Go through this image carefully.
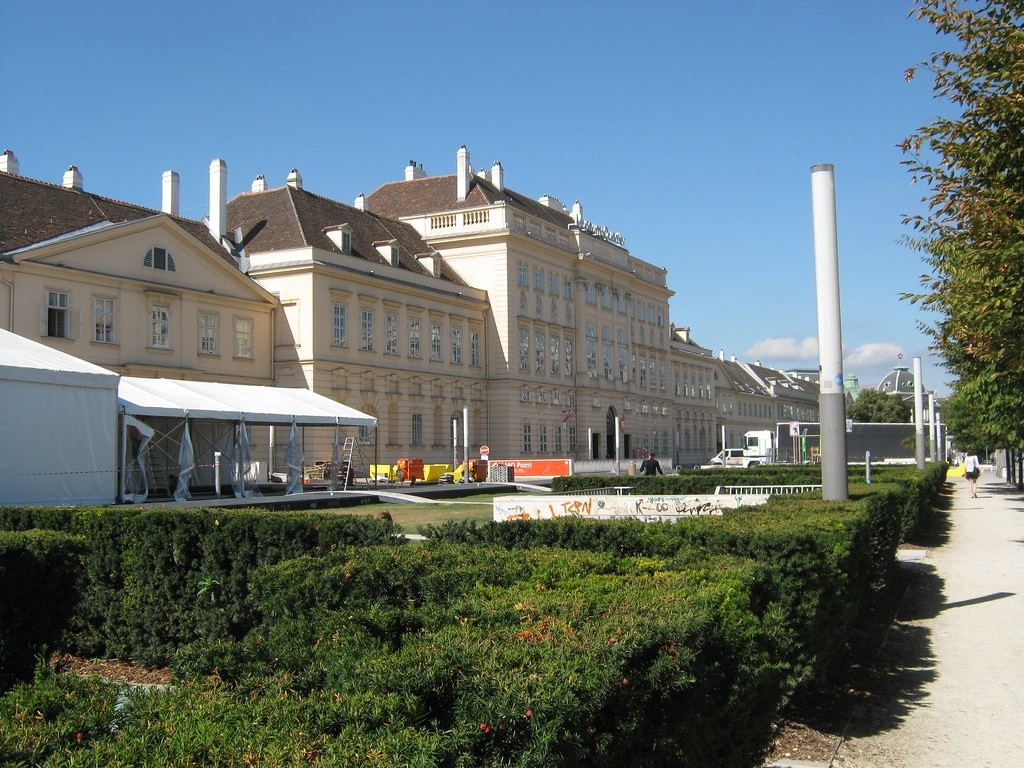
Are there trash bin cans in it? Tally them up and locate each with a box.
[628,459,637,476]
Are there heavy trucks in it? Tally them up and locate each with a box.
[741,419,947,471]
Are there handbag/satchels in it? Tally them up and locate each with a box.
[973,467,980,477]
[640,459,648,476]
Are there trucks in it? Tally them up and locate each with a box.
[463,458,573,484]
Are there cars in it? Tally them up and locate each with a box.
[438,474,454,484]
[708,448,743,465]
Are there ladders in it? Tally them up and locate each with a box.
[127,437,171,497]
[335,436,370,492]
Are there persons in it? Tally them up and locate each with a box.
[965,448,981,498]
[640,450,664,476]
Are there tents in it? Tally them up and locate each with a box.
[118,374,380,503]
[1,329,120,502]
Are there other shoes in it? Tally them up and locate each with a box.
[971,496,974,498]
[974,493,977,497]
